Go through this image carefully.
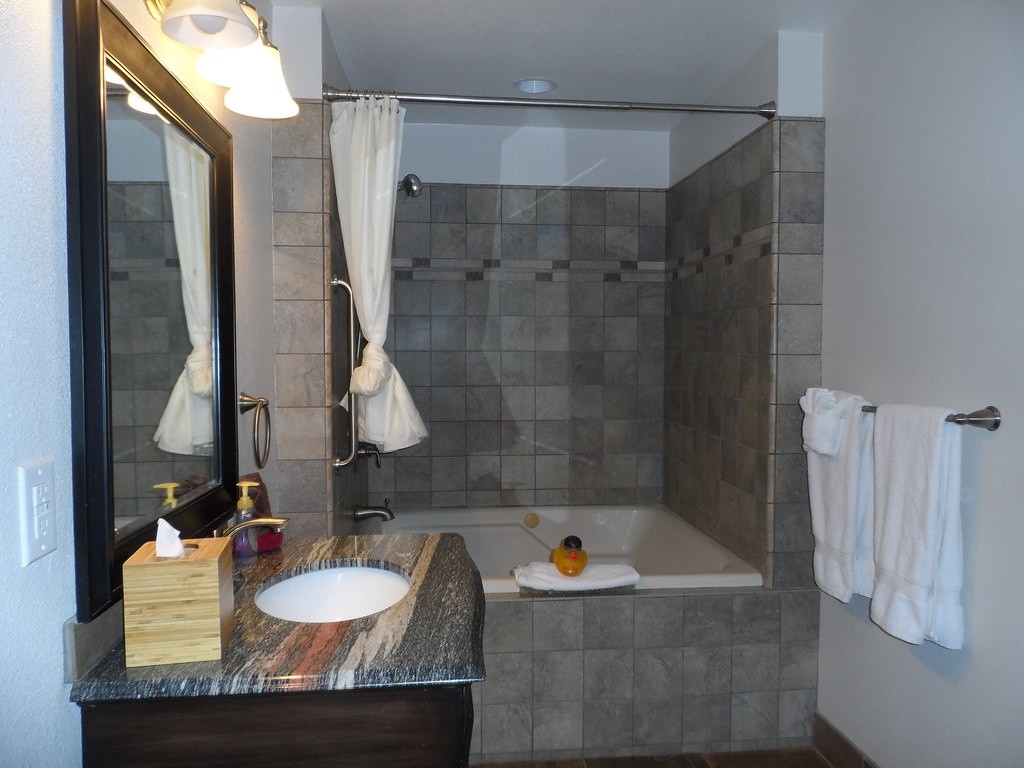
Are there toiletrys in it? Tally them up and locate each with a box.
[549,536,589,578]
[152,481,180,512]
[230,479,261,565]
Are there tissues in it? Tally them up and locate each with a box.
[122,517,235,669]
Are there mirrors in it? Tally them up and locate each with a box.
[61,0,239,629]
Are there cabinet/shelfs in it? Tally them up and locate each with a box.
[72,682,474,768]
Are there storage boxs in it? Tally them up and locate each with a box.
[122,538,233,669]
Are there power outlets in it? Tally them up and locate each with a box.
[18,455,59,567]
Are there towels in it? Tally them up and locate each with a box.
[511,560,642,592]
[796,386,968,653]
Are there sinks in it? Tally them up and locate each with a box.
[254,556,411,624]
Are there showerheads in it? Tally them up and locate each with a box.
[396,173,424,199]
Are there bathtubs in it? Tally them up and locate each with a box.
[533,503,763,590]
[353,504,554,592]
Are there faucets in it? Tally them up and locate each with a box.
[219,515,291,543]
[352,506,397,523]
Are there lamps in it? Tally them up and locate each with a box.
[222,16,300,121]
[160,0,259,49]
[194,0,270,90]
